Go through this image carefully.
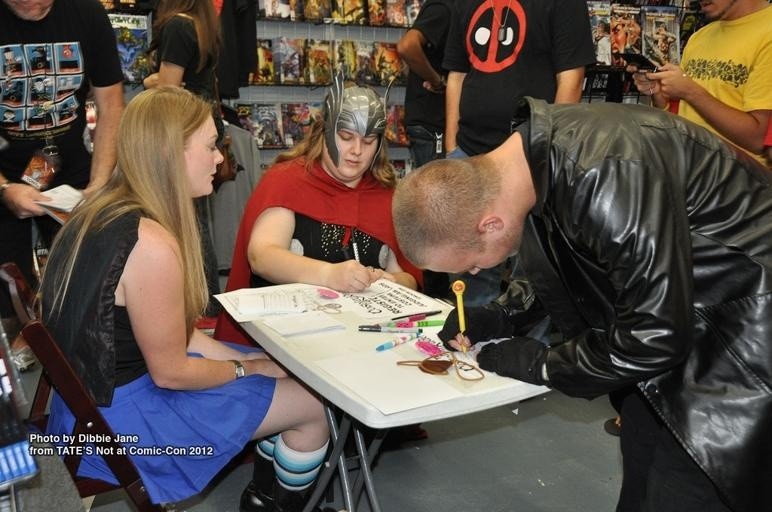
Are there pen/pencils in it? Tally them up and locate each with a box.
[452,280,467,356]
[376,320,445,327]
[376,333,419,352]
[353,242,361,263]
[395,314,425,323]
[392,310,442,321]
[358,325,423,333]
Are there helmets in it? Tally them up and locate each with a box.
[323,69,396,171]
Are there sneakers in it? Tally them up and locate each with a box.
[406,424,428,442]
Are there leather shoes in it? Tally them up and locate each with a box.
[238,480,274,512]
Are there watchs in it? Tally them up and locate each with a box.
[227,359,245,381]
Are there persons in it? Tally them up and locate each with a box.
[391,97,772,511]
[213,70,428,444]
[32,83,331,510]
[0,0,772,379]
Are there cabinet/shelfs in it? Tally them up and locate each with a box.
[85,0,711,151]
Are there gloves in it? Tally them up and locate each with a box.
[438,302,510,352]
[476,336,549,386]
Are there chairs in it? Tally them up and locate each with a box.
[0,262,253,512]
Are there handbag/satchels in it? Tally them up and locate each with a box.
[215,144,237,183]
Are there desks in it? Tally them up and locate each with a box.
[236,280,552,512]
